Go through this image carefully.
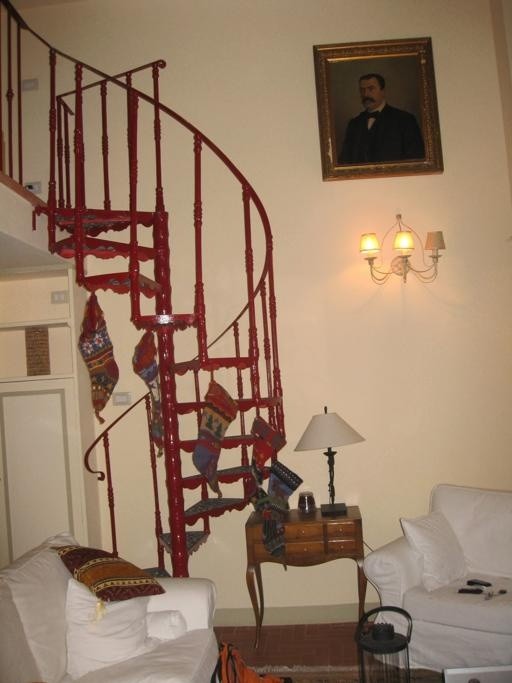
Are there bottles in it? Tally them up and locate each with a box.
[297,491,316,512]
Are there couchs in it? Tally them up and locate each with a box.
[363,484,512,673]
[0,531,220,683]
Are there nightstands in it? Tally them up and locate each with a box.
[246,506,367,647]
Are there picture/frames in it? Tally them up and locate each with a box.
[312,36,444,182]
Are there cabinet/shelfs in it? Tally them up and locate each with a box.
[0,262,102,567]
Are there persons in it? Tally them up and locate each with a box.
[342,74,426,164]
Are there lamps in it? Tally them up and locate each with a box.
[359,213,446,281]
[294,412,366,517]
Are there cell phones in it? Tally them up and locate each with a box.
[466,578,493,587]
[458,586,484,595]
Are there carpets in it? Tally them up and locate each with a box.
[245,667,442,681]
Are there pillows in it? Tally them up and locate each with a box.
[66,577,163,680]
[52,545,165,601]
[400,512,469,592]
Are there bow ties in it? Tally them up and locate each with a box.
[365,110,382,119]
